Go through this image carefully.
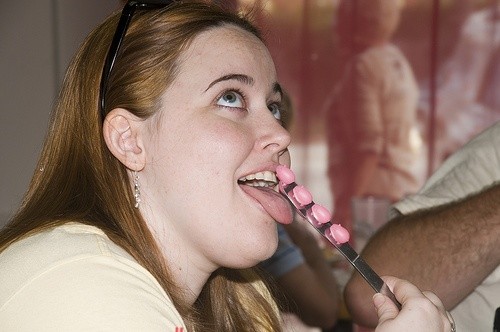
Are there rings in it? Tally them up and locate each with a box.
[450,322,456,332]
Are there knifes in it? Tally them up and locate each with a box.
[279,180,401,312]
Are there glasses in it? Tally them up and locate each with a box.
[99,0,175,128]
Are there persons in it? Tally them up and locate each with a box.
[343,117,500,332]
[251,89,345,330]
[1,1,458,332]
[322,0,430,280]
